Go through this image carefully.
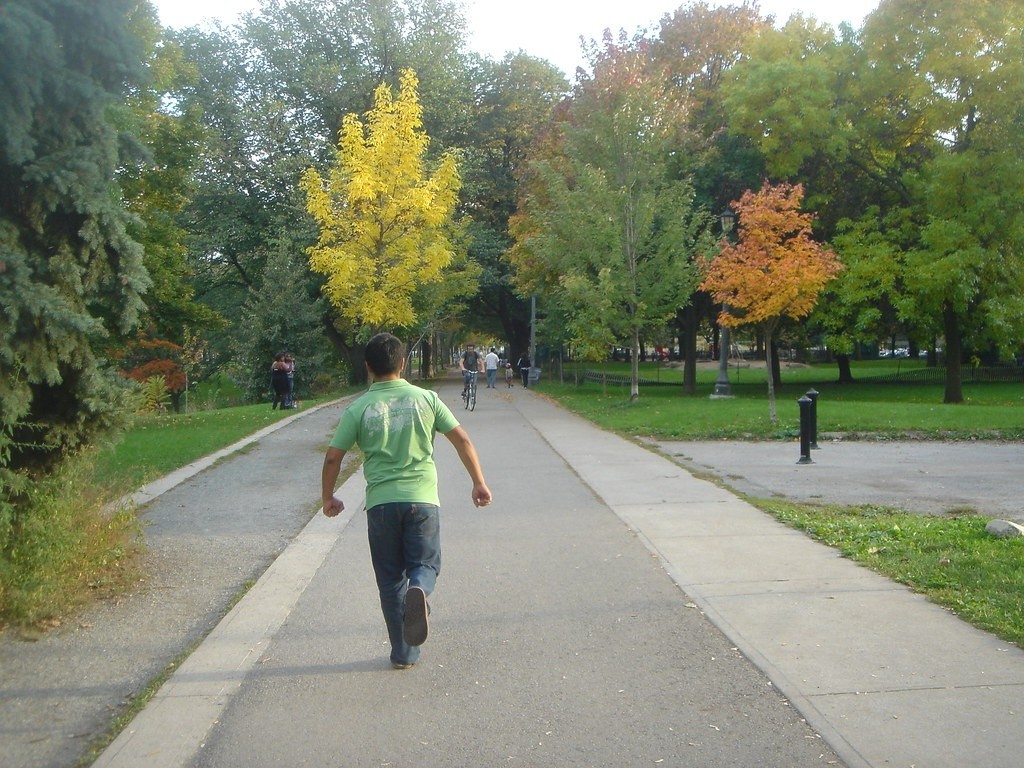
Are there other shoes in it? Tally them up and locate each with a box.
[389,650,412,669]
[400,585,431,647]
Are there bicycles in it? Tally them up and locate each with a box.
[462,370,479,411]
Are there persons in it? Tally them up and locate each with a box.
[453,351,459,366]
[484,347,500,388]
[271,352,296,410]
[459,344,484,403]
[517,352,531,389]
[321,332,493,669]
[506,363,514,388]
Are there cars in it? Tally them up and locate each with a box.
[880,348,927,357]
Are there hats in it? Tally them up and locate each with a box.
[274,352,284,361]
